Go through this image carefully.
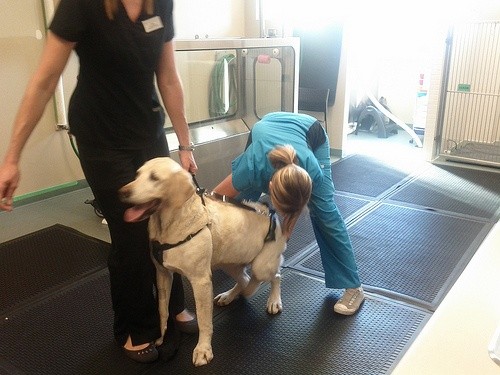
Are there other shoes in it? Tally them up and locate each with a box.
[121,341,161,364]
[172,315,199,336]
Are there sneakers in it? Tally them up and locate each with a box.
[333,287,366,316]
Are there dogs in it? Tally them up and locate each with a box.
[120,157,287,367]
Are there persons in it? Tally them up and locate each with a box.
[212,112,366,315]
[0,0,199,361]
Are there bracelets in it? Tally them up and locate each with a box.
[179,141,195,152]
